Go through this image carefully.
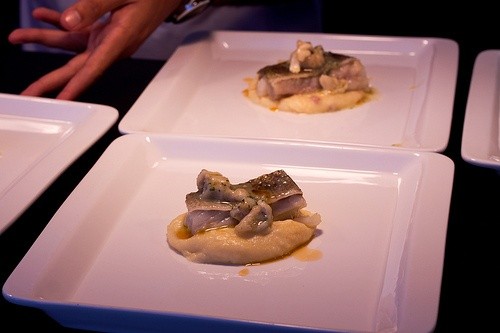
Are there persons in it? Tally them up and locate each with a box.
[7,0,180,101]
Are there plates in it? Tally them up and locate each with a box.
[460,50,500,169]
[2,133,455,333]
[0,93,120,235]
[117,29,459,153]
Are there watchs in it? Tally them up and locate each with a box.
[165,0,214,25]
